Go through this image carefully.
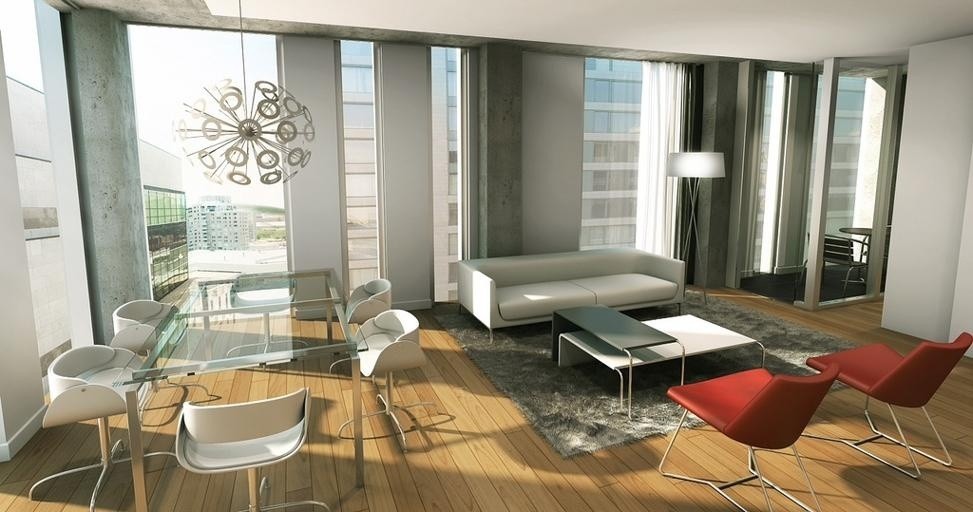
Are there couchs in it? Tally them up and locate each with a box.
[453,245,687,344]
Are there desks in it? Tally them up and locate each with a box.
[838,226,891,287]
[111,265,369,511]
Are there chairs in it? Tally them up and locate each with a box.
[332,308,441,454]
[221,272,309,370]
[655,359,843,512]
[27,344,178,511]
[168,386,337,512]
[797,330,972,482]
[326,278,392,387]
[109,298,223,428]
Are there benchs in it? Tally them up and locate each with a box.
[795,228,870,301]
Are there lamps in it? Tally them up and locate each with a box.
[665,150,729,304]
[173,0,321,192]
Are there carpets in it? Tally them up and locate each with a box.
[429,286,867,461]
